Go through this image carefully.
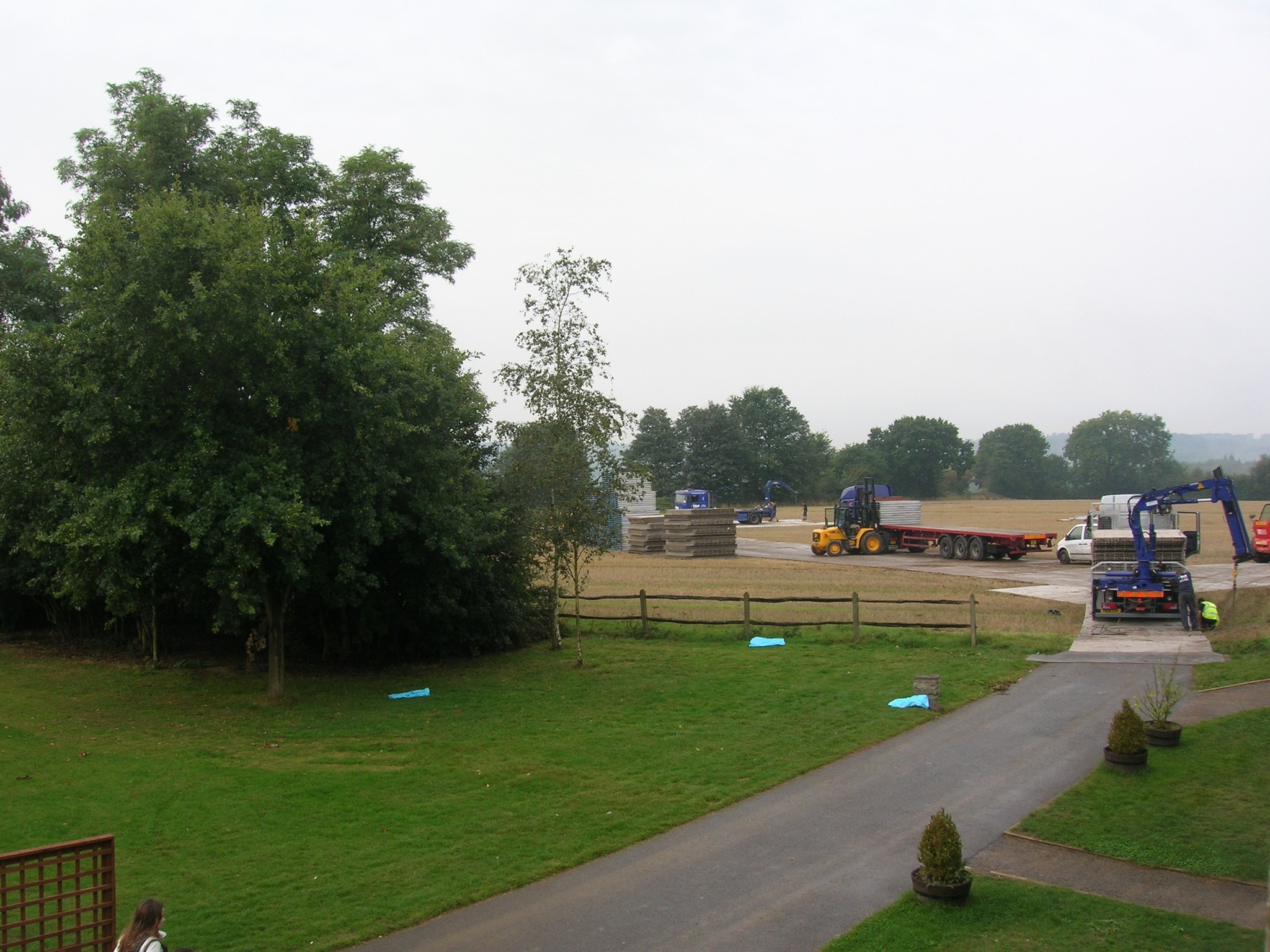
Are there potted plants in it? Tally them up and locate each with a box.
[1134,646,1183,747]
[1104,699,1148,770]
[911,807,973,898]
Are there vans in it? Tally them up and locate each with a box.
[1056,524,1096,564]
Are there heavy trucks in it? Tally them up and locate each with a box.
[834,484,1057,561]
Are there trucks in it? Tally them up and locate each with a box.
[1087,493,1200,621]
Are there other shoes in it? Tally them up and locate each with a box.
[1212,626,1217,631]
[1192,628,1204,631]
[1181,628,1188,631]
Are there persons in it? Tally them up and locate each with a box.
[1197,598,1219,631]
[800,501,808,520]
[768,502,779,522]
[1169,570,1201,632]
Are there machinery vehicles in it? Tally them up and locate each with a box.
[810,477,894,558]
[1092,466,1270,604]
[674,480,800,525]
